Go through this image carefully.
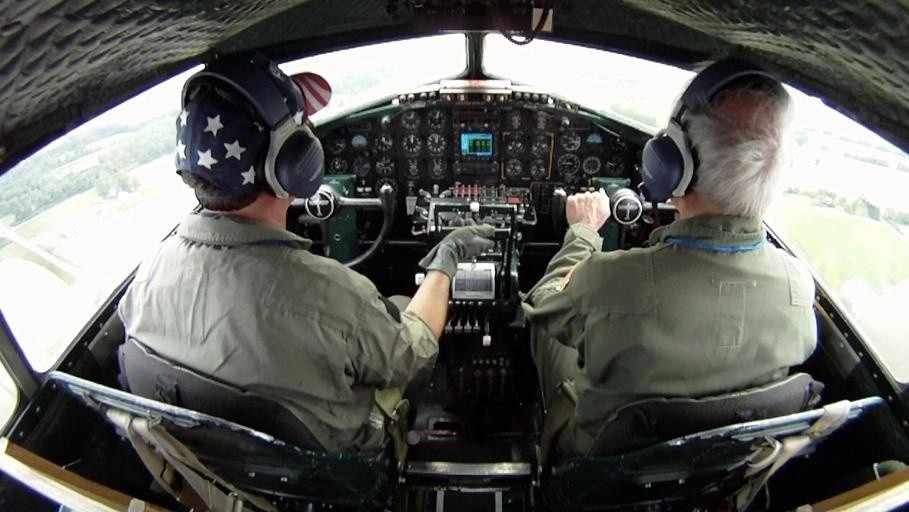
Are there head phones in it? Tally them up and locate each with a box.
[182,63,326,199]
[640,59,781,202]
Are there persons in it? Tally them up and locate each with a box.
[117,53,498,497]
[519,56,820,468]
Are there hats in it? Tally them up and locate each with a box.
[175,49,331,193]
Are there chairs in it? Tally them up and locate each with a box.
[519,335,821,473]
[117,334,413,473]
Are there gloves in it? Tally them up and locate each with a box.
[418,217,496,279]
